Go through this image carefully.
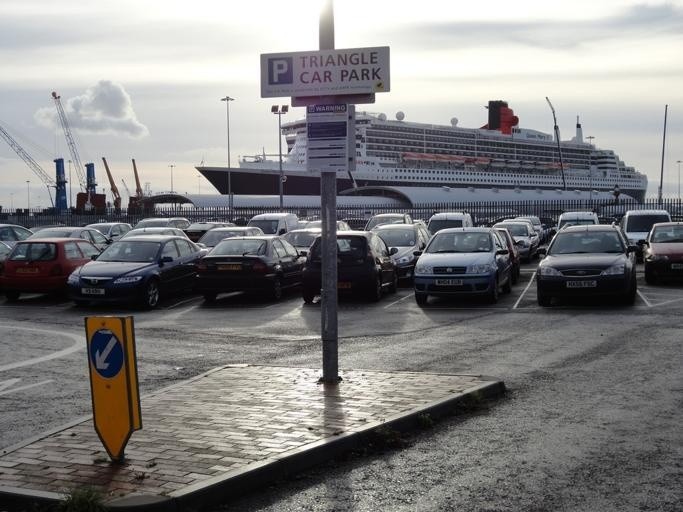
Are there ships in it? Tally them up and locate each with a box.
[193,98,650,211]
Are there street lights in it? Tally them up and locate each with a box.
[674,159,682,202]
[220,95,237,218]
[583,132,598,205]
[268,105,291,213]
